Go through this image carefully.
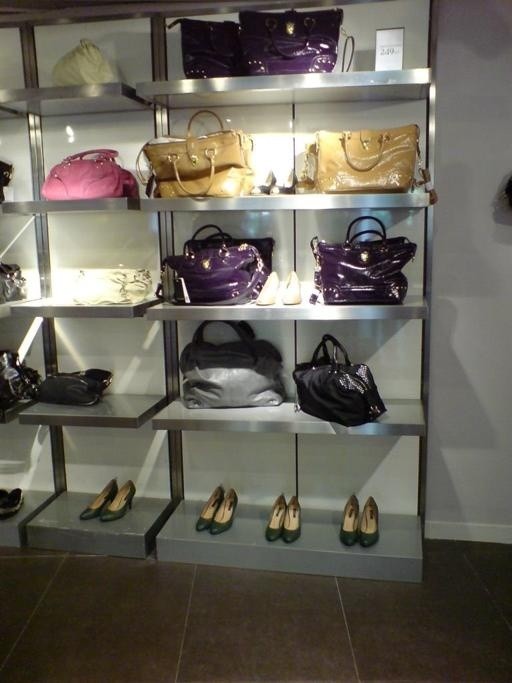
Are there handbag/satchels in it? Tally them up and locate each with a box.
[49,8,347,87]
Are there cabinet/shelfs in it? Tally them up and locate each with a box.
[154,1,435,582]
[1,0,162,560]
